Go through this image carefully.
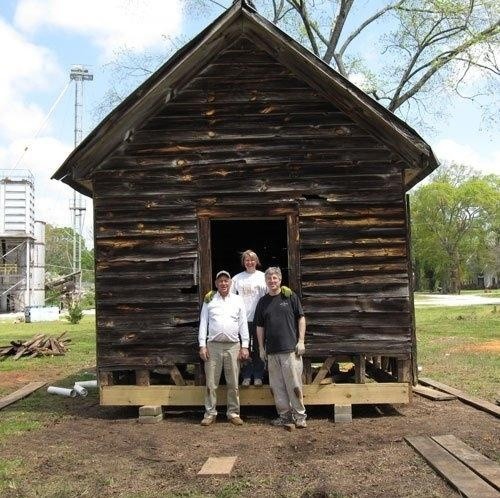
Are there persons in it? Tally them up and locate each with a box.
[252,266,308,429]
[203,249,293,388]
[197,270,251,425]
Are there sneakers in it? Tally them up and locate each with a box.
[228,417,244,425]
[254,379,263,387]
[295,419,307,428]
[242,378,251,387]
[272,417,289,426]
[201,415,216,426]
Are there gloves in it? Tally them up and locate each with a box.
[259,347,268,363]
[281,285,293,298]
[204,290,216,303]
[295,339,306,357]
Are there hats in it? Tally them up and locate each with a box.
[216,270,230,279]
